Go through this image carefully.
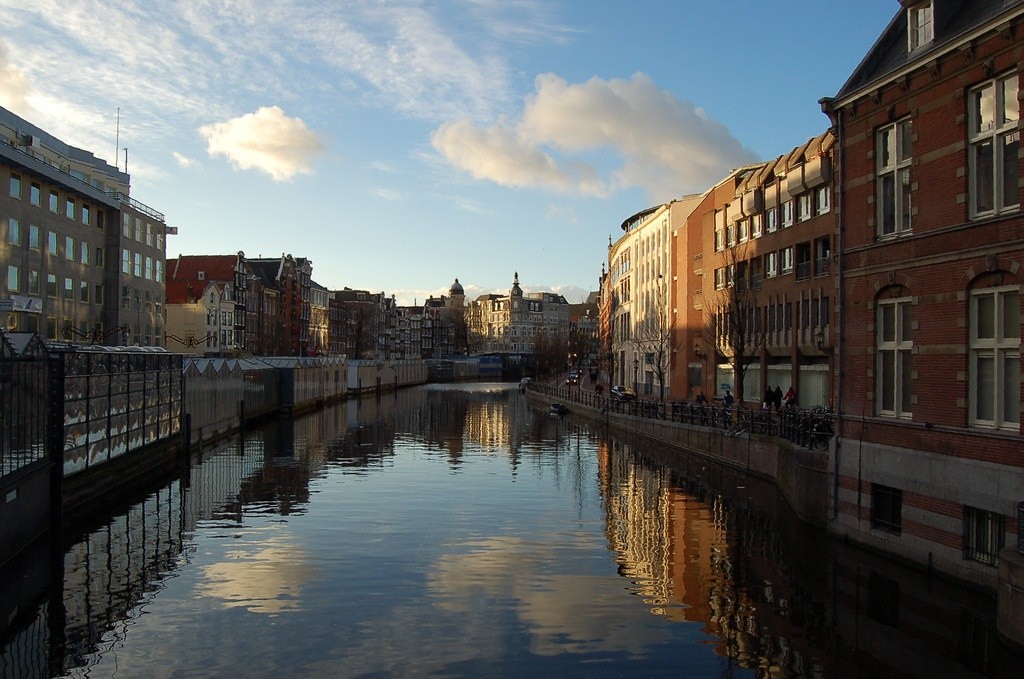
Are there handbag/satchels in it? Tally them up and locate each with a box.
[788,397,795,405]
[763,403,767,408]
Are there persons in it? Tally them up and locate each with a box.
[764,386,774,409]
[782,387,797,409]
[695,390,708,406]
[588,365,605,396]
[773,385,783,412]
[724,389,734,409]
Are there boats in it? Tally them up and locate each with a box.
[545,403,567,417]
[517,378,532,394]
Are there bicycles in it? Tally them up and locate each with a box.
[636,398,835,451]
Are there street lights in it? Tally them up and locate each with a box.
[633,359,639,403]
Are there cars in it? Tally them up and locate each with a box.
[611,386,634,399]
[566,378,577,385]
[570,373,578,379]
